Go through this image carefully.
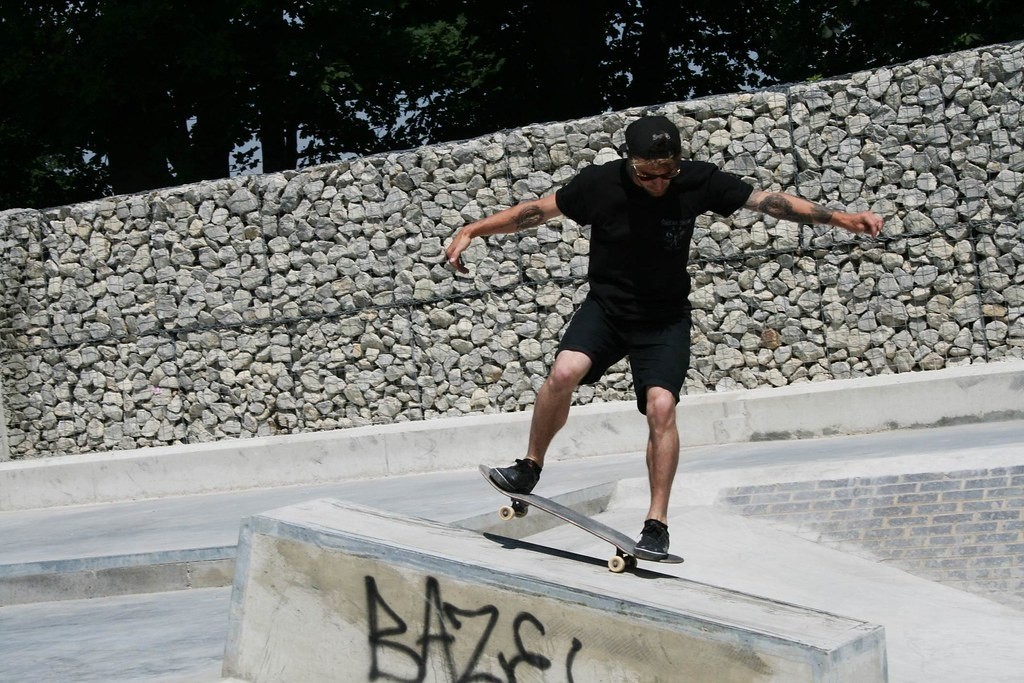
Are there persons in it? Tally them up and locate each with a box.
[446,115,883,561]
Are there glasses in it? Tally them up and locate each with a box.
[632,161,681,180]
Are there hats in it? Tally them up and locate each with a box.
[618,115,681,159]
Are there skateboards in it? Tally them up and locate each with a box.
[477,463,686,574]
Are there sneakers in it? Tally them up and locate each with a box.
[490,458,541,495]
[634,519,670,561]
